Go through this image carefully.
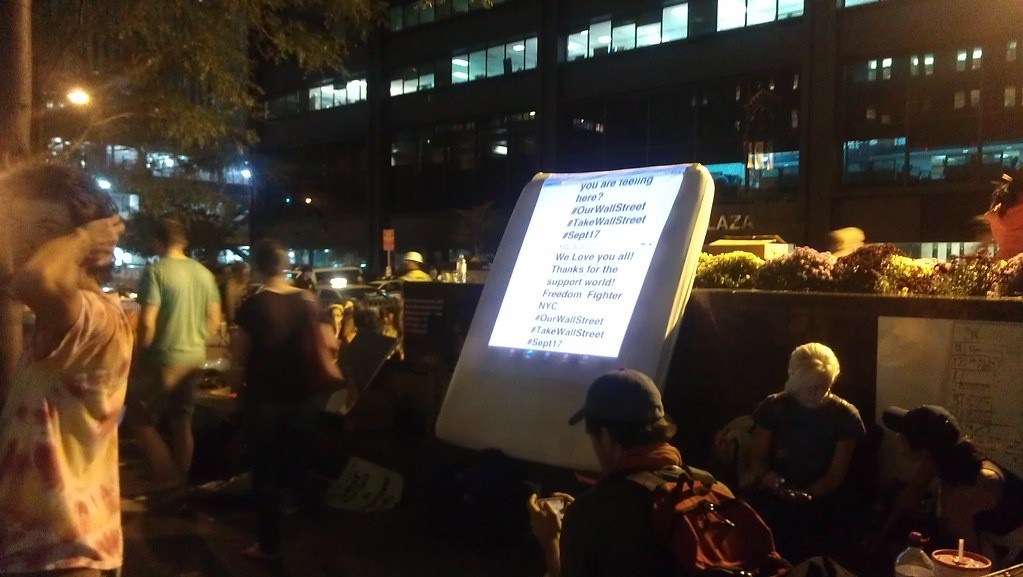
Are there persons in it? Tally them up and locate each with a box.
[232,243,324,557]
[528,371,734,576]
[223,251,436,434]
[0,163,131,577]
[984,174,1021,259]
[751,341,864,562]
[862,403,1021,570]
[136,217,221,512]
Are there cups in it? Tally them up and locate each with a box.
[930,548,991,576]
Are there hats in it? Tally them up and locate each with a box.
[883,404,961,445]
[568,367,664,428]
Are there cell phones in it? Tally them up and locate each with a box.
[536,497,565,521]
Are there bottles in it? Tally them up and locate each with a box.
[457,254,466,283]
[894,532,935,577]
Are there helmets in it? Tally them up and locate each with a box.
[403,251,424,264]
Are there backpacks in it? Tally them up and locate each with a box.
[628,464,795,577]
